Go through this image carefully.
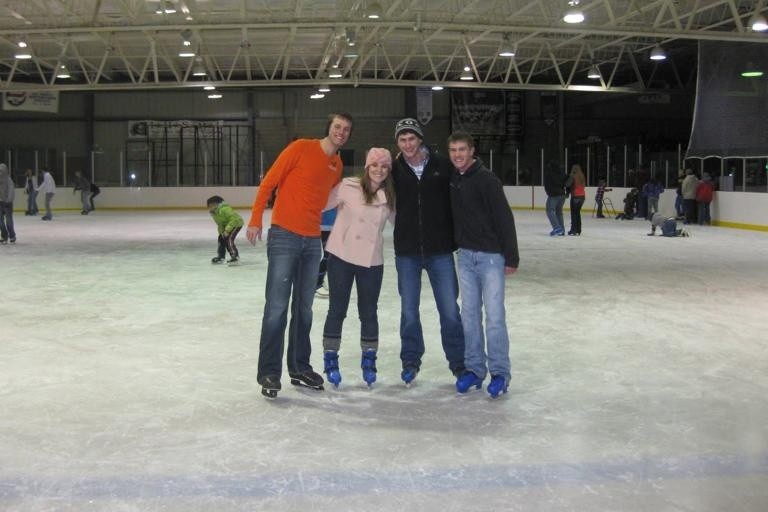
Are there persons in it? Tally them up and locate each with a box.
[23,169,38,215]
[36,169,57,221]
[323,147,396,386]
[89,181,102,211]
[0,163,16,245]
[439,131,520,397]
[380,117,466,384]
[246,112,353,391]
[205,195,243,265]
[73,170,90,216]
[544,158,713,237]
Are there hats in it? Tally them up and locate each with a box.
[365,148,392,169]
[395,118,423,139]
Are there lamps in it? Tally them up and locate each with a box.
[430,0,586,93]
[750,13,768,31]
[308,0,384,102]
[152,0,224,102]
[648,40,666,61]
[12,36,72,83]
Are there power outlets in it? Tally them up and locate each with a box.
[587,60,601,78]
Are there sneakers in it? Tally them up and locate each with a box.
[214,256,238,263]
[324,352,341,383]
[290,371,323,386]
[455,369,507,395]
[262,375,281,391]
[361,351,377,382]
[551,226,580,235]
[402,367,417,381]
